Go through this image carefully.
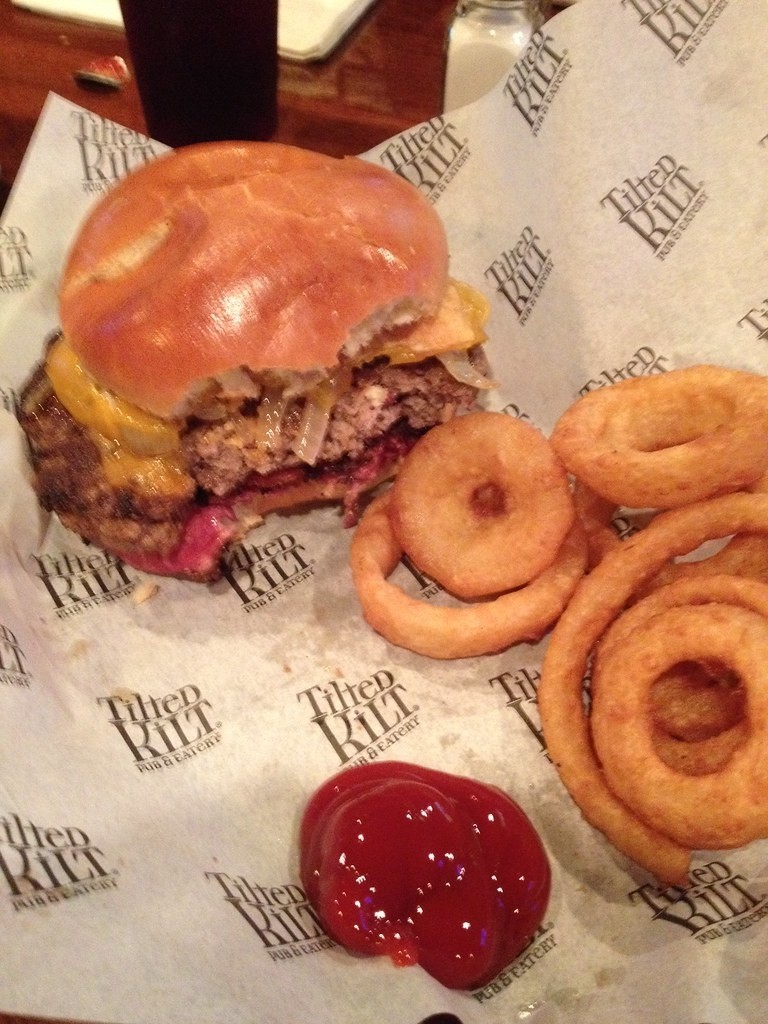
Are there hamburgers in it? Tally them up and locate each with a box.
[14,139,506,583]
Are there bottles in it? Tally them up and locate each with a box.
[442,0,545,118]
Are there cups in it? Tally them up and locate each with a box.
[119,0,278,150]
